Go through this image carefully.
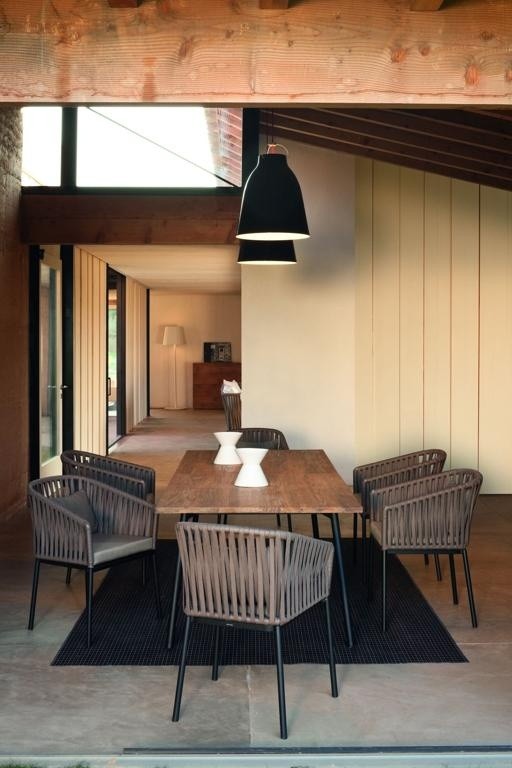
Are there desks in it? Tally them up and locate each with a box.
[155,450,364,648]
[193,362,241,409]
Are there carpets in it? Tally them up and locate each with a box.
[50,537,469,666]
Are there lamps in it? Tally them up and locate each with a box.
[235,108,311,242]
[163,325,190,411]
[236,241,298,266]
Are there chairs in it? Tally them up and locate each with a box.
[59,450,156,585]
[221,382,241,393]
[372,468,483,634]
[172,520,338,740]
[221,393,241,432]
[352,449,445,582]
[28,475,161,648]
[215,427,294,532]
